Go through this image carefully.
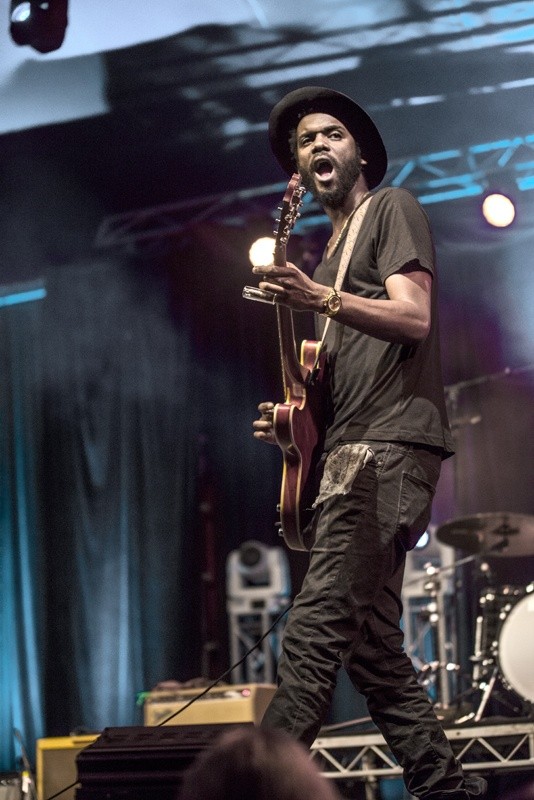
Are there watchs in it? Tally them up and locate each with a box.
[317,287,343,318]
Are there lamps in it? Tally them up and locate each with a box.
[231,224,277,268]
[475,169,529,229]
[5,0,69,55]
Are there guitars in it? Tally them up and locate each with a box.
[269,172,330,556]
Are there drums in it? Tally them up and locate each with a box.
[491,584,534,707]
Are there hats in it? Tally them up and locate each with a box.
[267,86,388,190]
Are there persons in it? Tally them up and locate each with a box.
[223,86,470,800]
[184,725,342,800]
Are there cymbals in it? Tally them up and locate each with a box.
[434,512,534,558]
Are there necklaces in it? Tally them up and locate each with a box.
[331,191,369,256]
